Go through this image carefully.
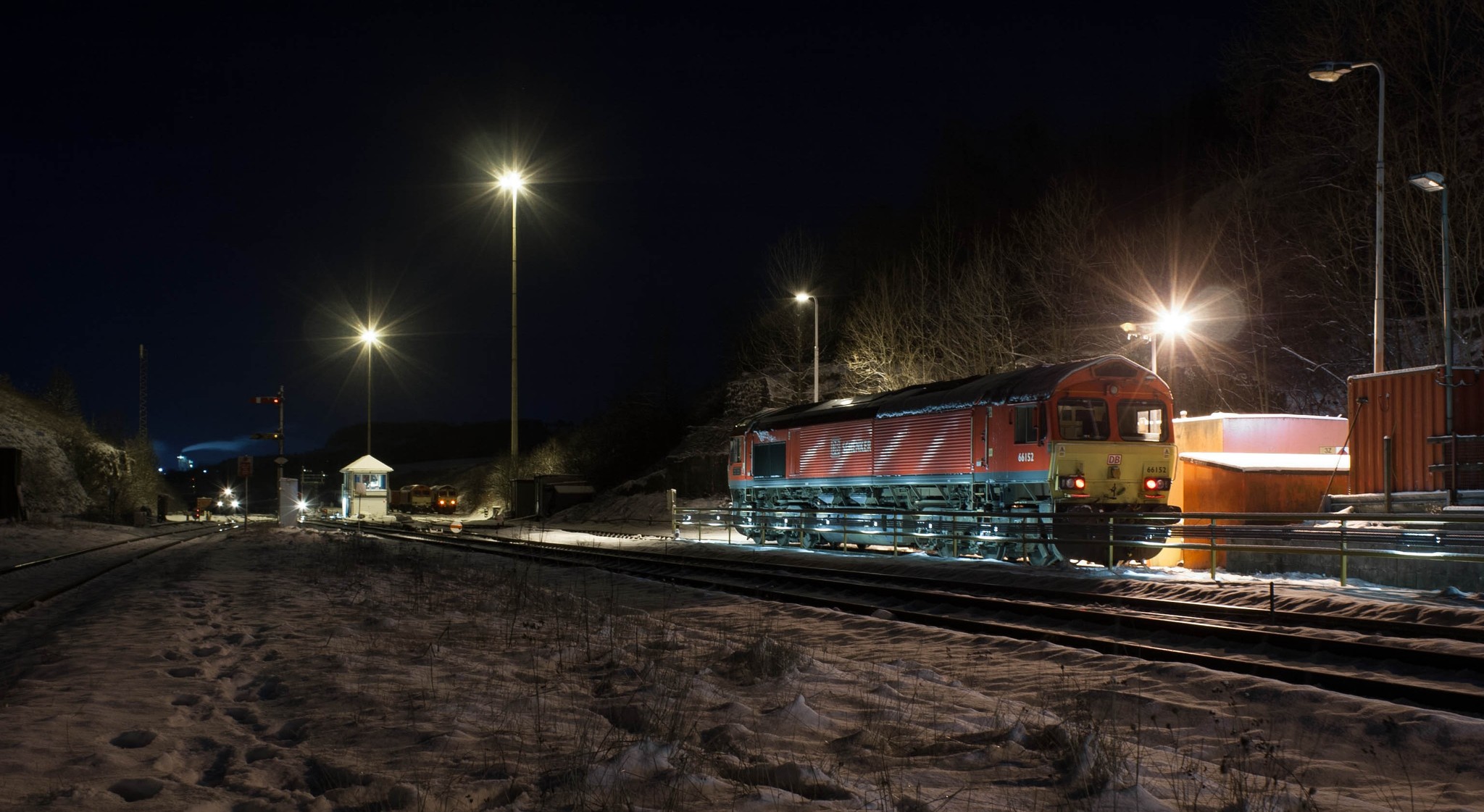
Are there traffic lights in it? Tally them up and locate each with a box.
[273,395,282,404]
[195,507,200,517]
[273,433,282,439]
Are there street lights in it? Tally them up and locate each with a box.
[1307,60,1387,370]
[1407,169,1460,508]
[495,170,524,458]
[361,328,378,454]
[795,292,818,403]
[1121,320,1157,374]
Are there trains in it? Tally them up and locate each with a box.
[211,486,241,514]
[430,484,457,514]
[388,484,433,514]
[726,348,1184,566]
